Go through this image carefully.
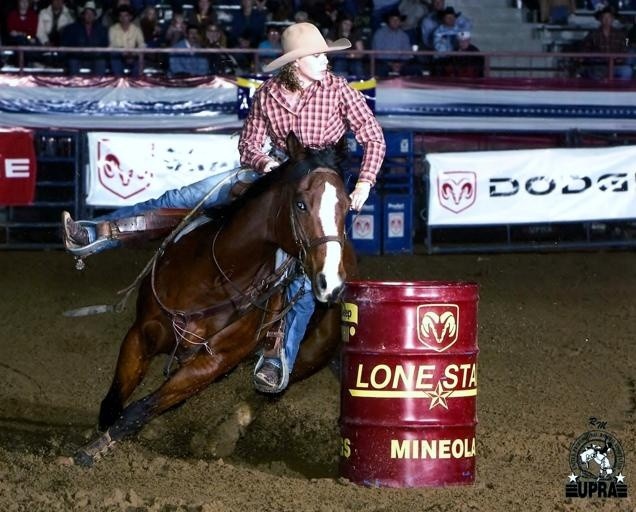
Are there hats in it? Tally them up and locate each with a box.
[261,23,351,73]
[437,6,462,20]
[381,6,407,23]
[456,31,471,41]
[594,4,624,21]
[77,1,102,19]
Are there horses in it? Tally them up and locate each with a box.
[72,129,361,474]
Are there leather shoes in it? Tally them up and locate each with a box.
[253,364,280,388]
[62,210,90,248]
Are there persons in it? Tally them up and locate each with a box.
[0,1,634,85]
[60,21,387,391]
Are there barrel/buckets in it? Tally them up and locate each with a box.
[339,278,479,488]
